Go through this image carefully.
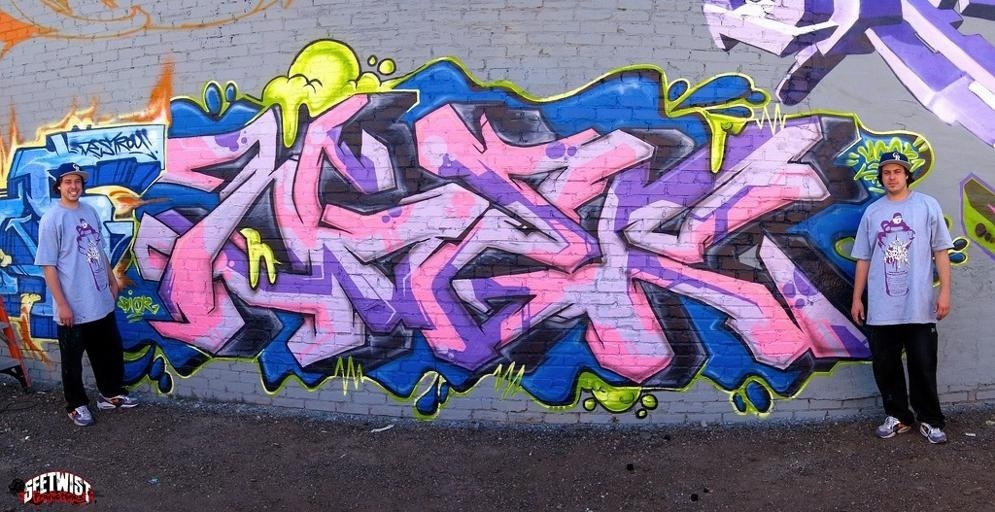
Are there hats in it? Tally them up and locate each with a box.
[55,162,89,180]
[878,151,912,171]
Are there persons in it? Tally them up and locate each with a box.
[850,151,955,444]
[32,161,142,428]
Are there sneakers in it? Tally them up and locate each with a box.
[96,392,142,410]
[919,421,947,445]
[874,415,912,439]
[67,404,96,427]
